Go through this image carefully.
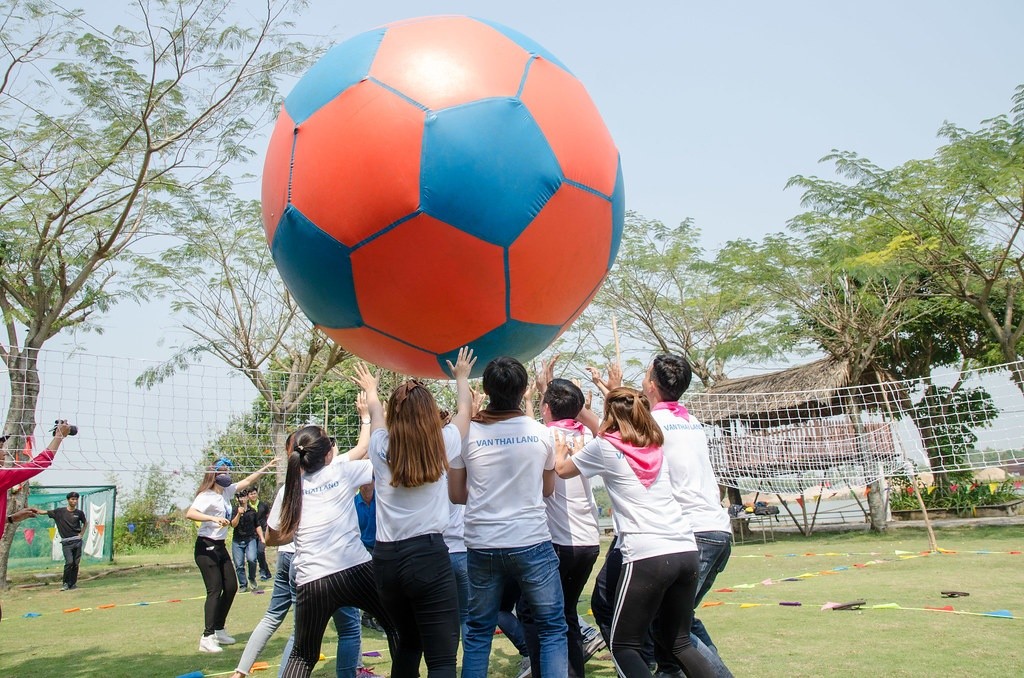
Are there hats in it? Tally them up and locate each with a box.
[0,434,11,442]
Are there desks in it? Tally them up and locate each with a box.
[730,514,776,545]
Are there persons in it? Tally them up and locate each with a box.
[247,487,270,581]
[230,487,266,593]
[37,492,88,590]
[0,419,71,541]
[233,346,733,678]
[186,458,282,653]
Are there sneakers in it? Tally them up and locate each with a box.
[199,635,223,652]
[215,629,236,644]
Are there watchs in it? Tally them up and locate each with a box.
[361,418,371,424]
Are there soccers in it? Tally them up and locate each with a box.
[260,13,627,381]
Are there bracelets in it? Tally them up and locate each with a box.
[8,515,13,523]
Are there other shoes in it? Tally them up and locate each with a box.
[69,585,78,590]
[249,579,258,590]
[515,656,531,678]
[238,587,247,593]
[60,583,69,591]
[260,571,267,579]
[582,631,607,663]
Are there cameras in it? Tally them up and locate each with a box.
[53,420,78,436]
